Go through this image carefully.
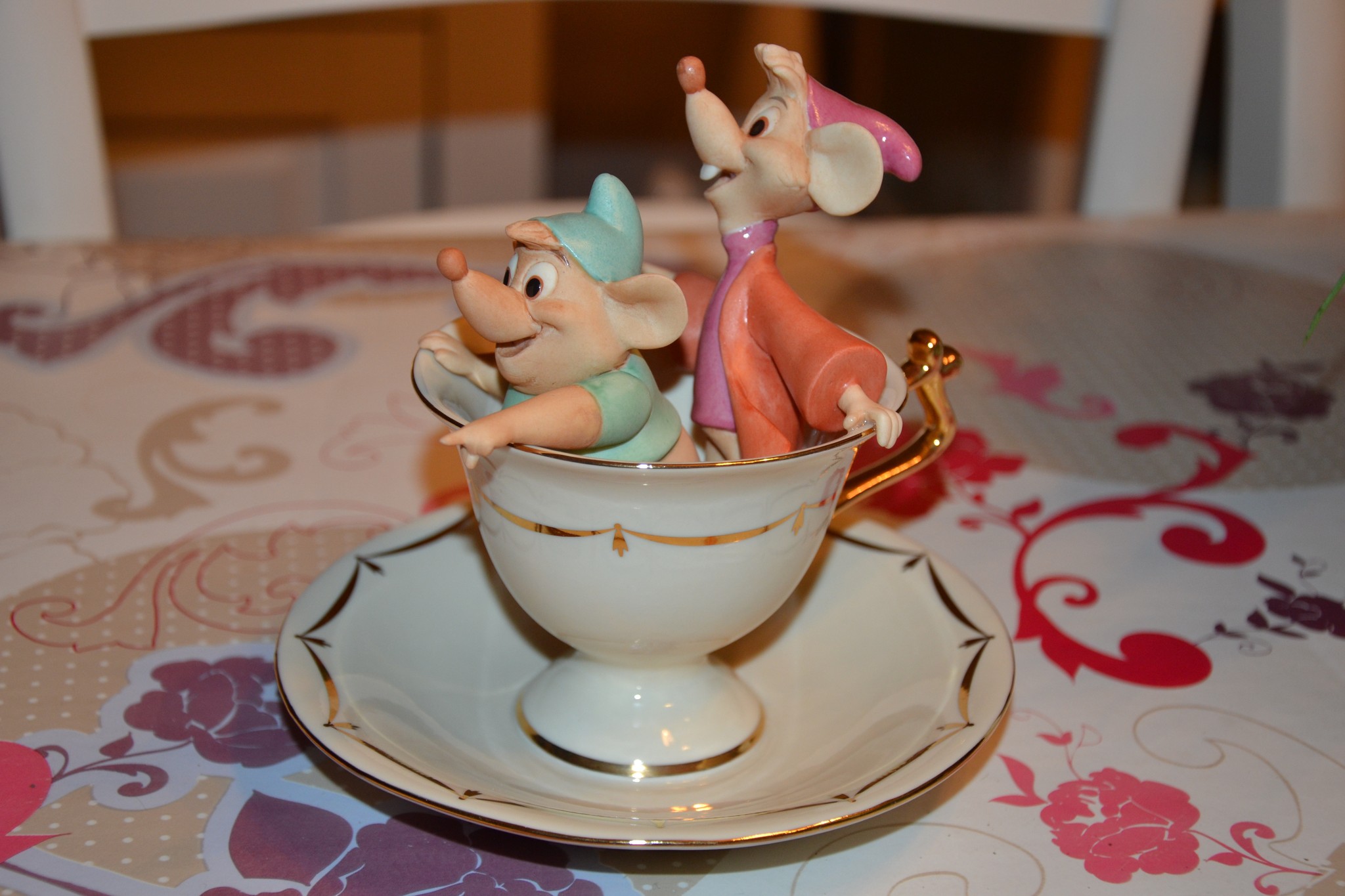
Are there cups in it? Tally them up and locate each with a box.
[414,302,912,778]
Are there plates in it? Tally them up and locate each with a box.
[275,492,1018,854]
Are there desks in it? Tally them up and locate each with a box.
[0,201,1345,895]
